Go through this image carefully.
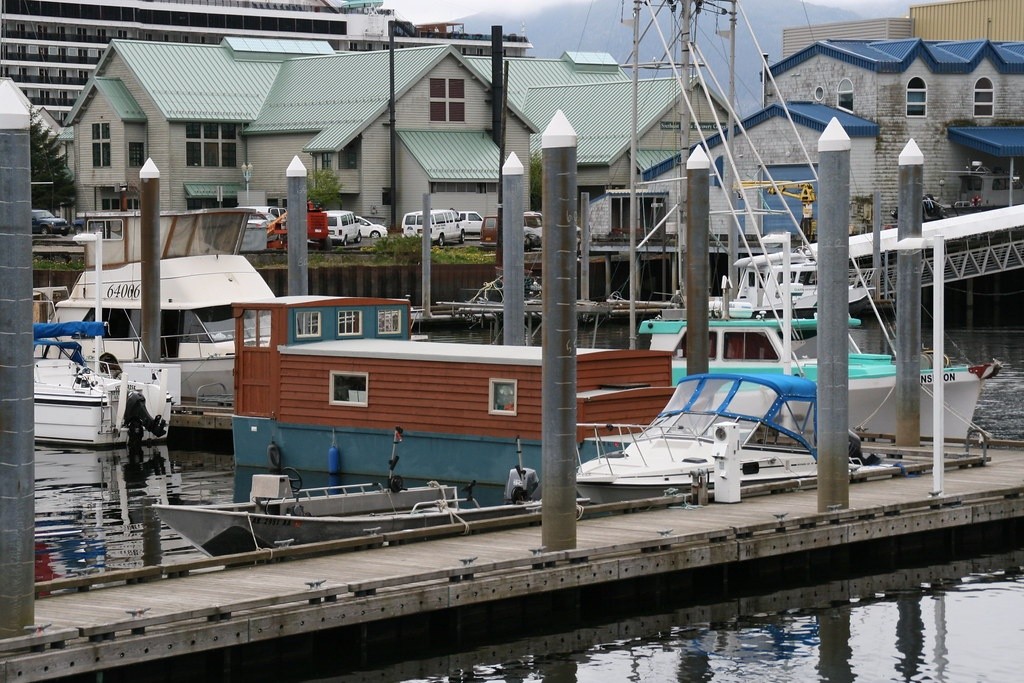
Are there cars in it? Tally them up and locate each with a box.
[459,211,483,234]
[481,212,581,253]
[355,216,388,238]
[33,210,70,236]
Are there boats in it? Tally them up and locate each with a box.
[232,0,1003,511]
[574,372,881,514]
[152,425,592,567]
[34,158,428,447]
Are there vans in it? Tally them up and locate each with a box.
[235,206,286,230]
[401,210,465,248]
[311,211,362,246]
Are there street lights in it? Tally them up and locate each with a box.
[242,162,253,206]
